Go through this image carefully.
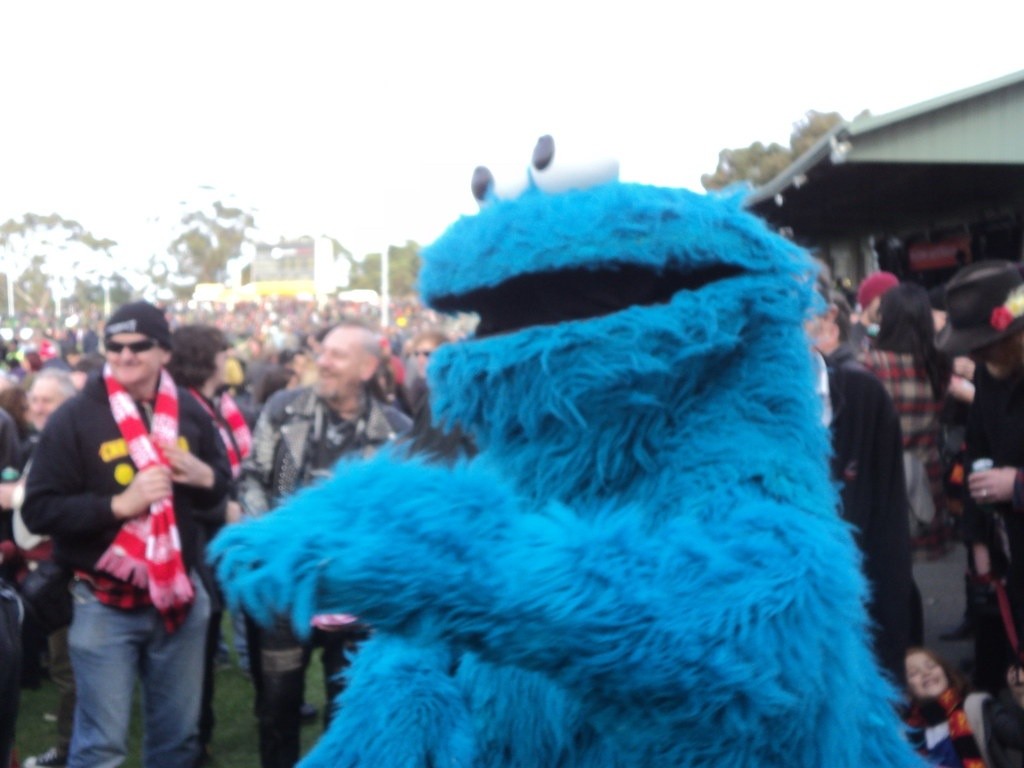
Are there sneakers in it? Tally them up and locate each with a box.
[22,746,57,768]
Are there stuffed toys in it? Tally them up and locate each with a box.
[206,133,925,768]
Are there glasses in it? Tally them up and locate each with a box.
[414,350,430,356]
[106,338,156,352]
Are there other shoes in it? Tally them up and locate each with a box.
[927,541,955,559]
[254,698,320,722]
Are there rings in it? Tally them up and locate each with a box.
[982,490,988,497]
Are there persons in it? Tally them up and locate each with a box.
[807,261,1023,694]
[901,645,1024,768]
[1,301,122,768]
[159,298,481,768]
[23,301,233,768]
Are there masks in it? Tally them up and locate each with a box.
[220,358,243,386]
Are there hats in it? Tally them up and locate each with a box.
[337,289,381,307]
[858,272,900,309]
[932,259,1024,354]
[102,301,176,349]
